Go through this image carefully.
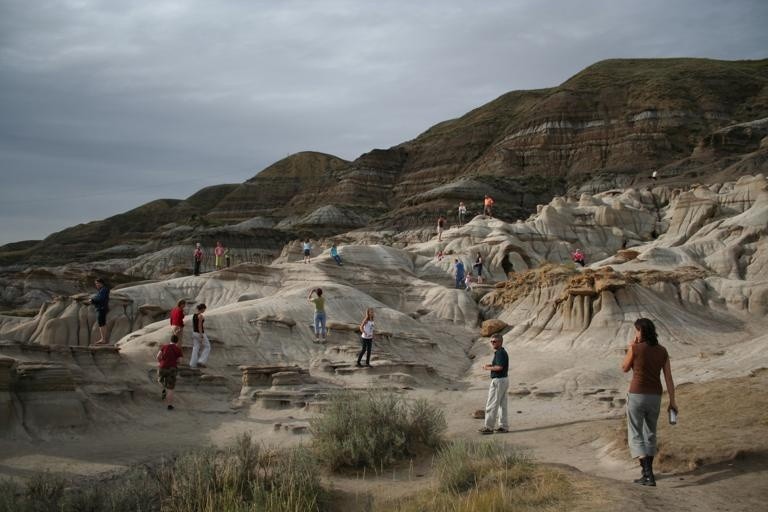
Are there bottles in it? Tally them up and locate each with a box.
[668,408,677,425]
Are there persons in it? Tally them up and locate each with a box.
[89,278,110,344]
[622,317,678,487]
[477,333,509,435]
[437,194,494,291]
[355,308,375,368]
[652,169,658,186]
[331,245,344,266]
[573,248,585,267]
[307,288,326,344]
[155,296,211,412]
[304,237,312,264]
[192,237,231,276]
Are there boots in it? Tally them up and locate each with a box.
[633,456,656,486]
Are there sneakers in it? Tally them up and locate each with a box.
[478,426,509,434]
[312,339,327,345]
[161,388,175,410]
